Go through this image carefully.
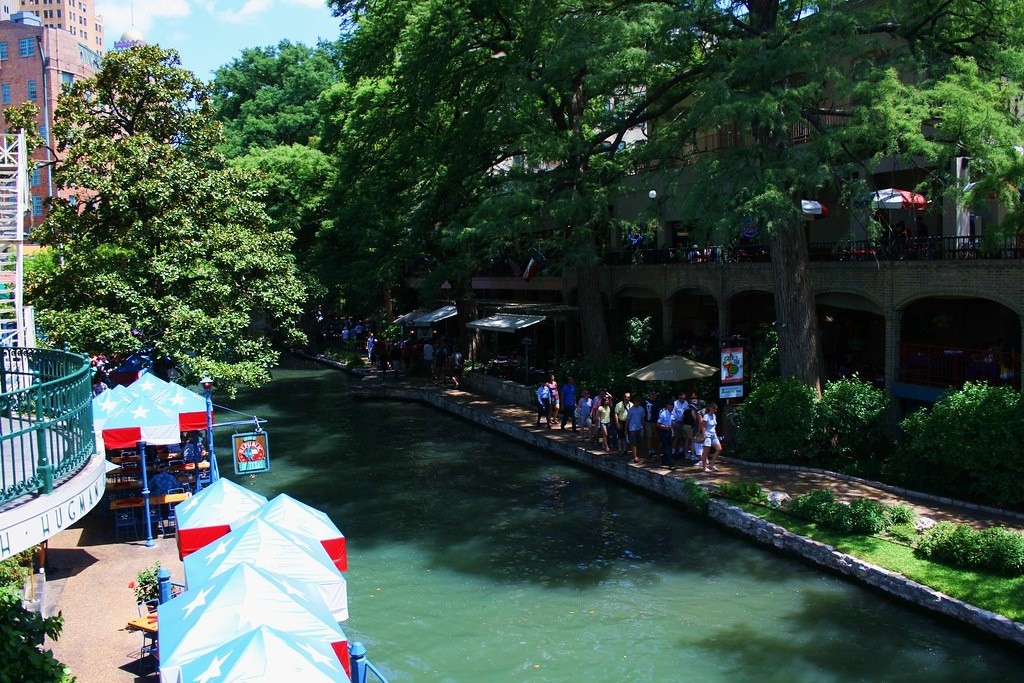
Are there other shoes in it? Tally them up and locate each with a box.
[553,419,559,423]
[546,424,551,429]
[618,450,627,457]
[561,424,565,429]
[693,459,709,466]
[572,427,577,432]
[648,448,656,457]
[685,453,698,460]
[603,446,610,452]
[702,466,712,472]
[549,421,555,424]
[659,465,676,470]
[634,457,639,462]
[537,421,540,427]
[708,466,718,472]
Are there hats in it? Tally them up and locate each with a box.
[687,403,699,410]
[157,462,169,471]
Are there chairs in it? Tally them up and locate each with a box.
[195,462,211,493]
[168,488,185,515]
[114,503,176,543]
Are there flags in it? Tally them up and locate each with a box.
[521,251,546,281]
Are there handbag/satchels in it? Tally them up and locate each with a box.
[694,433,704,443]
[574,407,578,418]
[544,398,549,403]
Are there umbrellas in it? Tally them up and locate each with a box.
[627,354,720,396]
[864,188,927,257]
[734,191,830,220]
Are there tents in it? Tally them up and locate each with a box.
[155,477,353,683]
[93,371,213,448]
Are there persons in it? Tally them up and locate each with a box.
[558,376,578,431]
[506,346,524,382]
[876,216,928,258]
[573,390,710,467]
[655,239,766,263]
[84,349,127,397]
[693,402,722,472]
[943,339,1017,386]
[533,373,560,429]
[325,318,371,352]
[363,332,463,389]
[143,429,208,519]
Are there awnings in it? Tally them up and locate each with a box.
[412,304,457,327]
[464,312,547,333]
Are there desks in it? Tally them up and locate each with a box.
[108,462,209,486]
[109,450,211,462]
[109,493,186,509]
